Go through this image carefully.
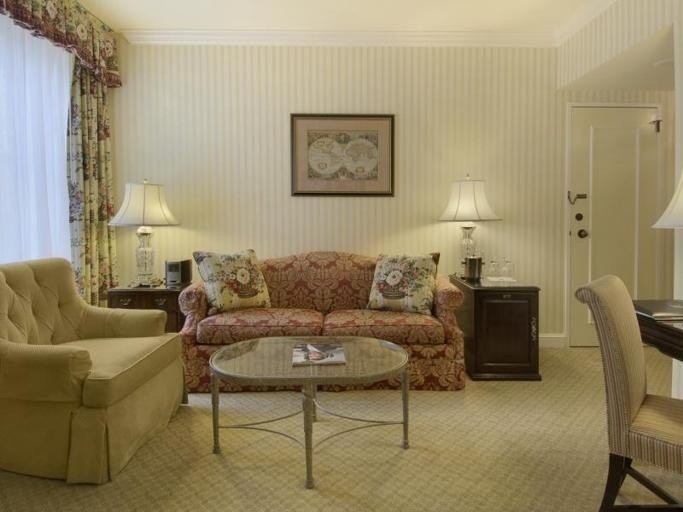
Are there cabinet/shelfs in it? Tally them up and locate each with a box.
[107,288,183,333]
[449,275,542,381]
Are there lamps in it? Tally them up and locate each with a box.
[651,171,683,229]
[108,178,182,286]
[437,174,502,279]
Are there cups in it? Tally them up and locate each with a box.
[485,260,500,282]
[499,259,516,283]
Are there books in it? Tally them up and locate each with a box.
[291,343,347,365]
[631,298,683,322]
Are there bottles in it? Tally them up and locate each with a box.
[461,253,484,283]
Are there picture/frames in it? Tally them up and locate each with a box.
[290,113,395,196]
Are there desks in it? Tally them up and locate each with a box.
[636,314,683,361]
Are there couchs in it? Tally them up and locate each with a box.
[179,251,466,394]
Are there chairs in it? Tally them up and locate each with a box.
[575,274,683,512]
[0,258,188,485]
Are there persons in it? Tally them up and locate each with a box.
[300,343,343,362]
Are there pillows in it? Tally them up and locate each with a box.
[366,252,440,315]
[192,248,272,315]
